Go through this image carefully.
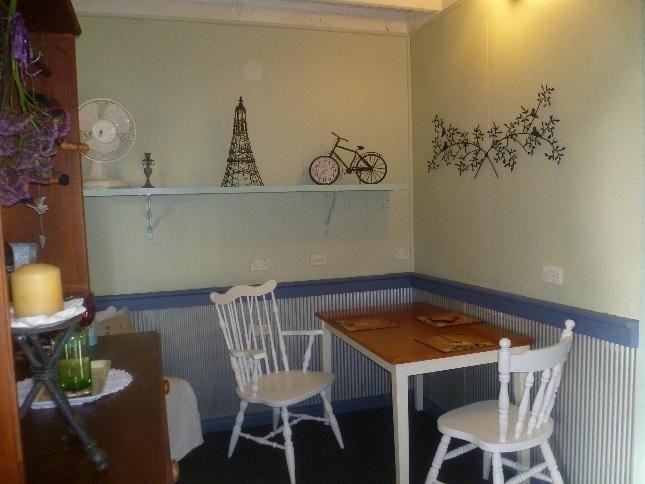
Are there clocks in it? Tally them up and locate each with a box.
[310,131,388,185]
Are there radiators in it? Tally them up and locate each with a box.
[90,270,641,483]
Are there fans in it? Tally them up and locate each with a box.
[74,96,138,189]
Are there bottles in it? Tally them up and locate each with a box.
[3,240,17,306]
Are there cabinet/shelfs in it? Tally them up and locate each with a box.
[0,0,184,483]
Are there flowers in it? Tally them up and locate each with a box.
[0,0,73,209]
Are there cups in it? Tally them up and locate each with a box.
[56,329,93,391]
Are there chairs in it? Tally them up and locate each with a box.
[421,319,581,482]
[203,278,348,483]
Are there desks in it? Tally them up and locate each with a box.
[310,299,541,483]
